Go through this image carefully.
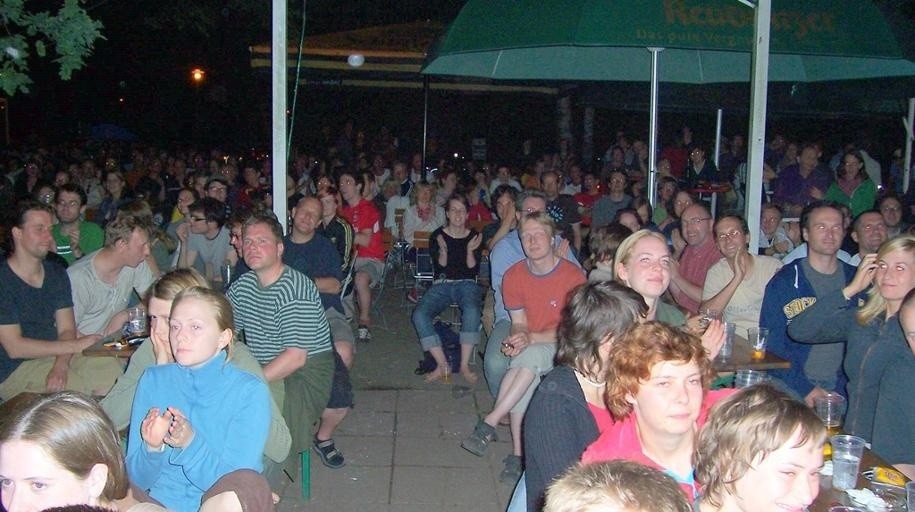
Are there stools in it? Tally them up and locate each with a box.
[302,448,311,497]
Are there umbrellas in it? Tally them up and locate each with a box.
[416,1,915,223]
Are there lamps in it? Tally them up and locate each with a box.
[190,68,204,80]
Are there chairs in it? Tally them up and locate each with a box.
[342,208,490,366]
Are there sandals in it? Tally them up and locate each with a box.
[312,432,345,468]
[461,366,478,383]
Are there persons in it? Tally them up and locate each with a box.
[315,121,457,303]
[1,138,383,512]
[411,119,915,512]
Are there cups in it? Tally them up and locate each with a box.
[815,390,849,459]
[828,432,867,491]
[828,470,914,512]
[126,306,148,337]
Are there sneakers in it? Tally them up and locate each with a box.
[357,324,370,343]
[408,287,419,303]
[461,418,498,458]
[498,454,524,485]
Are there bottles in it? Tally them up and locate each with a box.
[691,307,771,393]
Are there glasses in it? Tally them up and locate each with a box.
[190,215,205,222]
[679,217,711,228]
[518,207,537,214]
[208,186,225,193]
